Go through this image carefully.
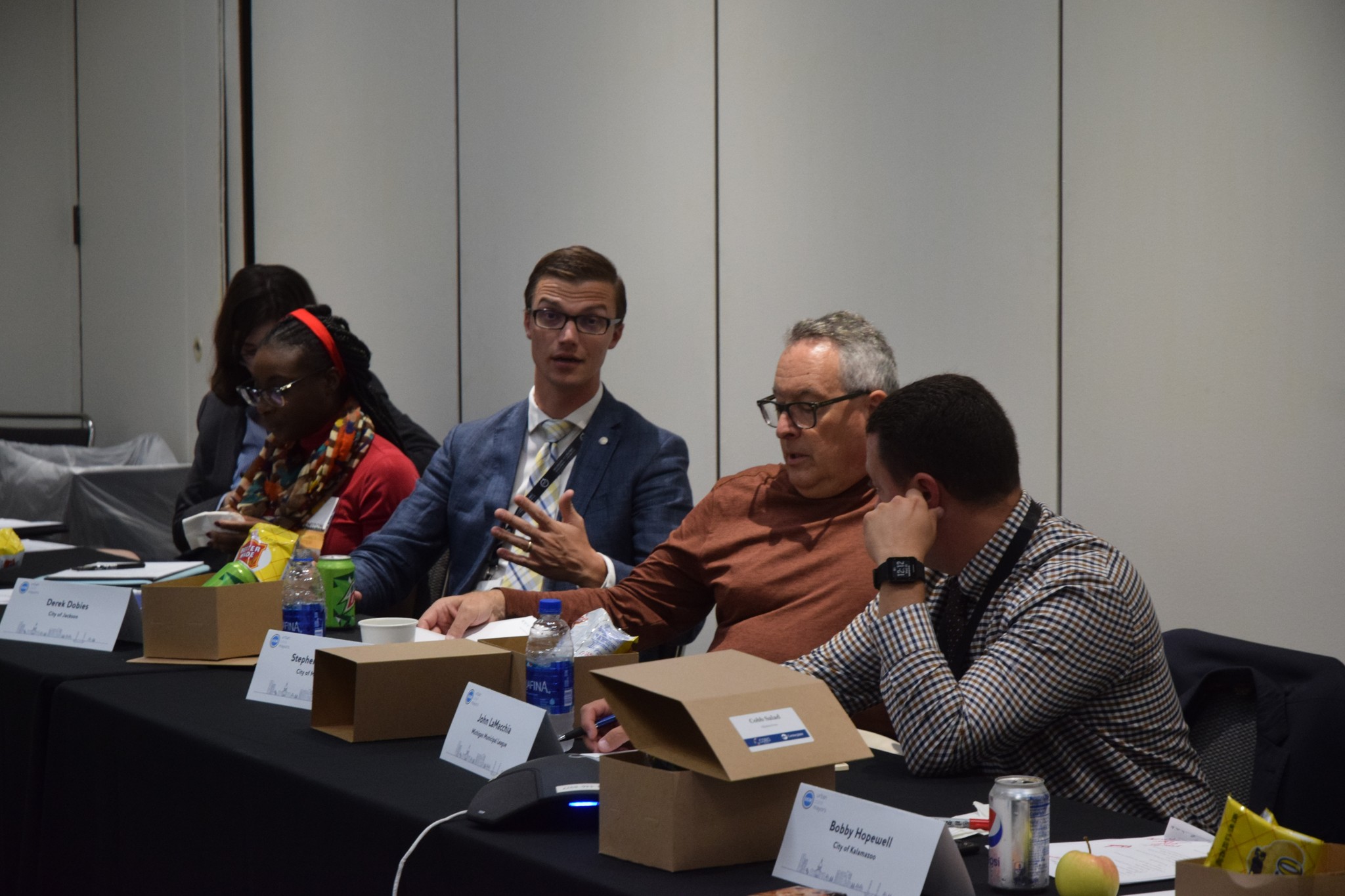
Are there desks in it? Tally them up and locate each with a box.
[0,517,1188,896]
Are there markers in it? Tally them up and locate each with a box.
[923,817,989,830]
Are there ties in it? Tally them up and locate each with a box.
[503,418,575,591]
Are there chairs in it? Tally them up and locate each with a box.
[1159,628,1345,845]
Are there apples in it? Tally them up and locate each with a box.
[1055,837,1119,896]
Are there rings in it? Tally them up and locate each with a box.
[524,540,532,552]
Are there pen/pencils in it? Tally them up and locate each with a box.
[72,562,145,570]
[558,715,618,742]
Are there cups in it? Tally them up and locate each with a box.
[357,617,419,644]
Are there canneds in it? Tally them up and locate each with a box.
[202,559,261,587]
[314,554,356,628]
[988,776,1050,891]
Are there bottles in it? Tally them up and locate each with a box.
[524,598,574,753]
[282,548,327,637]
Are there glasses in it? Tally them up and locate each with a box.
[236,373,313,411]
[756,388,872,430]
[530,308,622,335]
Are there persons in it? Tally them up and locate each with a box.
[207,302,420,619]
[292,245,696,615]
[418,311,900,736]
[580,375,1220,841]
[172,264,441,557]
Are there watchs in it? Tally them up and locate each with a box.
[872,556,926,589]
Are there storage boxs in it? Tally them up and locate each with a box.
[1175,846,1345,896]
[477,636,638,734]
[141,572,286,660]
[599,751,836,871]
